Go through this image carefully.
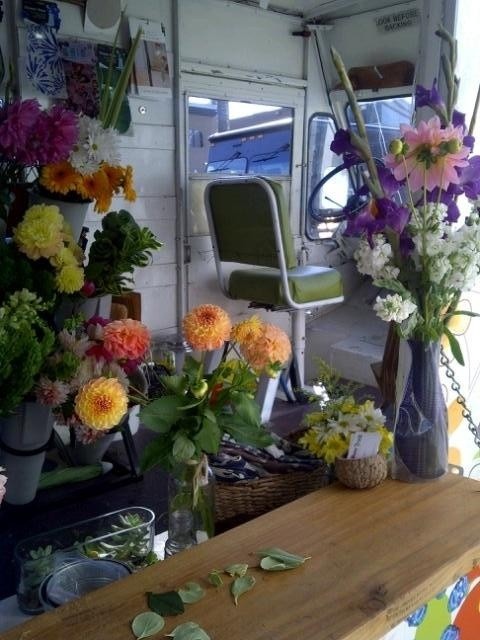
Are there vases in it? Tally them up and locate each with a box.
[392,339,452,482]
[1,399,52,505]
[164,456,216,561]
[331,452,389,494]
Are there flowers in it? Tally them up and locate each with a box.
[324,21,478,363]
[1,89,163,444]
[77,303,296,489]
[303,387,395,467]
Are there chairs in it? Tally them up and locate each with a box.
[203,174,347,404]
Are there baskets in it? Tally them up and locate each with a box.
[206,462,331,527]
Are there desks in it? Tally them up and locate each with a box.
[1,471,479,640]
